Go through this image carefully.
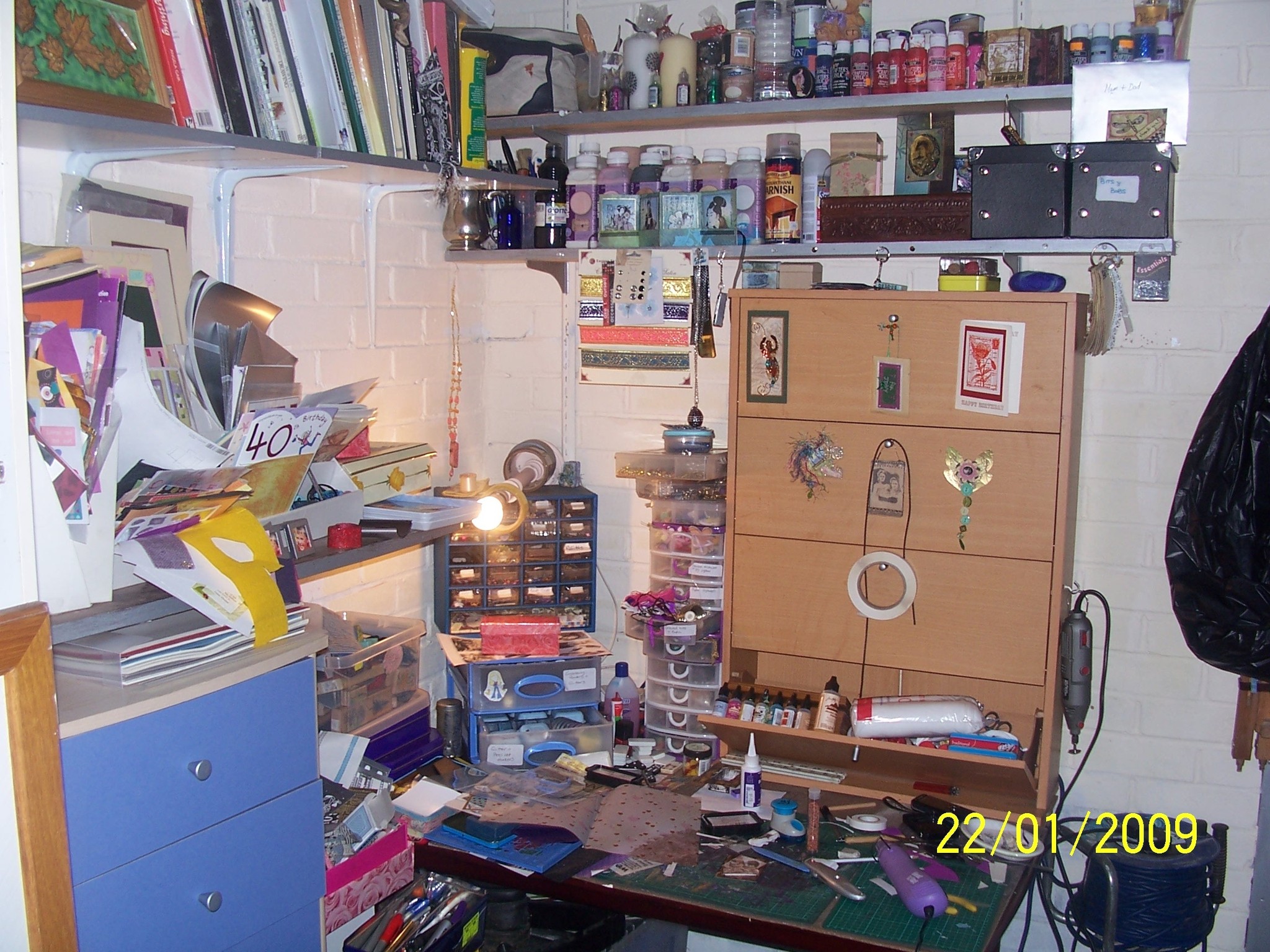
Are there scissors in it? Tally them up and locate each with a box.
[984,711,1012,734]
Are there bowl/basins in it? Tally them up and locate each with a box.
[662,429,715,454]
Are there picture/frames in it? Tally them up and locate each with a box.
[265,518,315,561]
[14,0,175,123]
[894,111,956,193]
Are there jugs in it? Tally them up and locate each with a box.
[575,51,623,114]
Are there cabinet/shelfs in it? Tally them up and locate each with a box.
[447,632,613,767]
[57,658,328,952]
[433,482,598,635]
[440,84,1176,294]
[696,288,1090,829]
[625,521,730,764]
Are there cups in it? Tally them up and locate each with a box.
[948,12,986,47]
[911,18,946,34]
[875,29,911,39]
[436,698,463,758]
[442,188,509,251]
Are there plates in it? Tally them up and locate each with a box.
[960,818,1044,861]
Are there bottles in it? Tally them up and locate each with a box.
[752,689,771,724]
[497,193,523,250]
[740,732,763,807]
[648,75,663,108]
[603,661,640,745]
[767,692,785,726]
[779,694,799,728]
[610,79,624,110]
[676,69,691,106]
[793,694,812,729]
[712,681,730,718]
[1070,21,1175,66]
[533,132,832,250]
[813,675,840,733]
[738,687,756,721]
[724,685,742,720]
[814,30,985,98]
[706,69,720,104]
[719,0,871,104]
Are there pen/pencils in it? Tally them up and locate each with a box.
[354,877,490,952]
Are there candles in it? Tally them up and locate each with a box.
[328,522,362,550]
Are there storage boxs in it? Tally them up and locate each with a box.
[829,131,884,196]
[480,615,561,654]
[967,143,1071,240]
[742,261,822,288]
[314,609,486,952]
[1067,141,1179,238]
[940,254,1002,293]
[258,457,363,546]
[820,192,972,242]
[614,448,728,526]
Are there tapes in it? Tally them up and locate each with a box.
[846,813,887,831]
[847,550,918,620]
[480,483,528,535]
[503,440,556,493]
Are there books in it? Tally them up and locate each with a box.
[148,0,462,166]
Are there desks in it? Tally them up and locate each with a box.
[389,756,1041,952]
